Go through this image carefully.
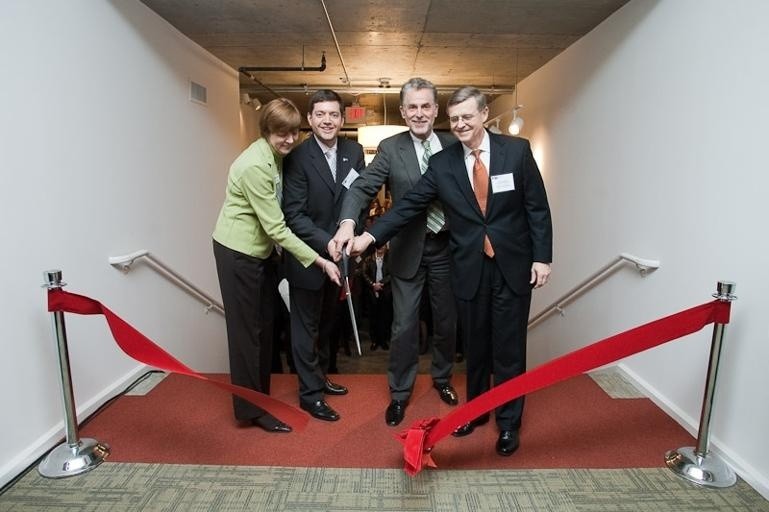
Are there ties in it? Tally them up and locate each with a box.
[471,149,495,258]
[420,139,445,235]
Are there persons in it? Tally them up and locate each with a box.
[349,87,553,457]
[281,88,369,422]
[419,289,429,355]
[332,78,459,427]
[363,241,393,351]
[212,94,344,433]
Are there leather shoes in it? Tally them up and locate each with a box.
[496,428,519,456]
[369,343,378,351]
[432,381,458,405]
[247,412,292,433]
[385,399,408,425]
[453,415,489,436]
[323,379,348,395]
[380,342,389,350]
[299,399,339,421]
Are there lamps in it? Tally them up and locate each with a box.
[483,103,527,138]
[241,91,263,113]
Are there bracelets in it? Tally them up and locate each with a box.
[322,259,327,273]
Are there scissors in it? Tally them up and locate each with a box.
[337,243,362,356]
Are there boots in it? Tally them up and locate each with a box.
[418,321,429,354]
